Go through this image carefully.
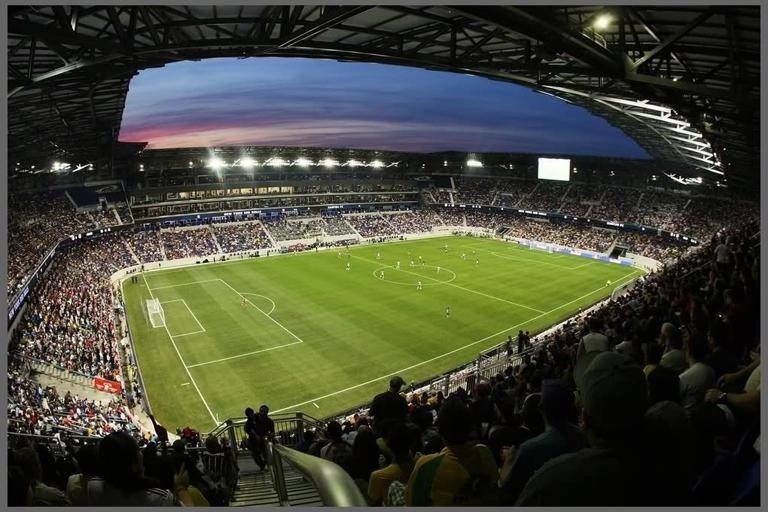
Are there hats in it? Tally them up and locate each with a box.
[390,376,406,386]
[573,350,648,429]
